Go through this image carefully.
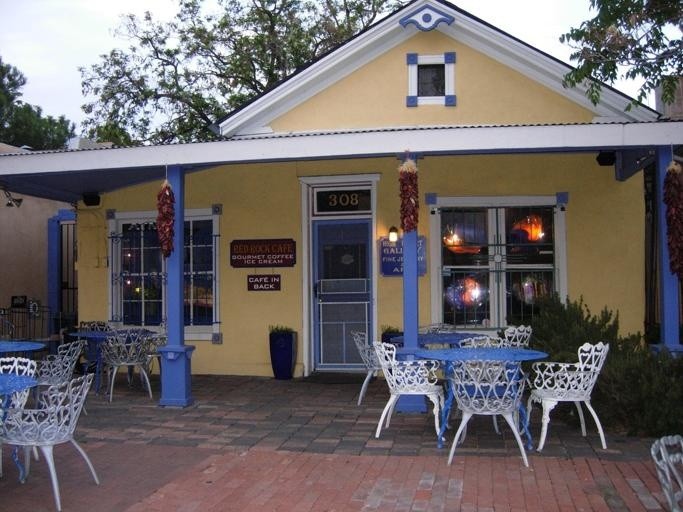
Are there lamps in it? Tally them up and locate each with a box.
[388,226,399,242]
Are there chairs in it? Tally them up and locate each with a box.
[0,321,168,511]
[349,323,609,467]
[648,433,683,512]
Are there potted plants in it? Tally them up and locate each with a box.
[268,323,297,381]
